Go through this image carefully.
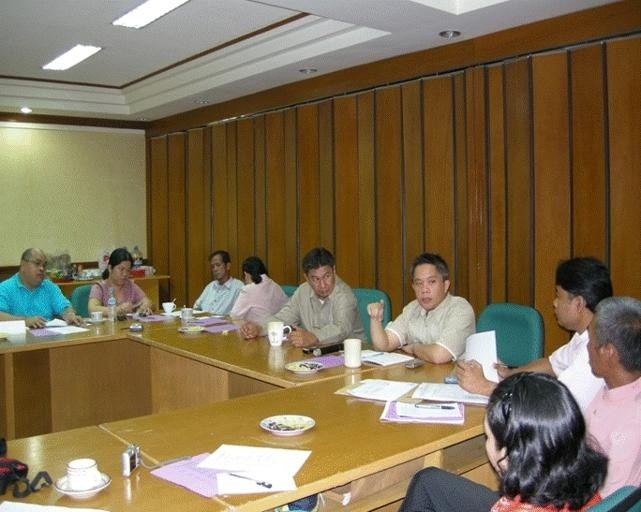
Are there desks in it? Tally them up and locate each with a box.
[0,307,499,512]
[52,273,171,311]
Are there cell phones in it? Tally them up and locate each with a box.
[303,347,323,353]
[404,358,424,368]
[445,377,458,383]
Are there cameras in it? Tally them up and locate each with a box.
[129,323,144,331]
[121,443,140,476]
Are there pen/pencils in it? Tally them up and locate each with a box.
[362,352,386,358]
[502,364,518,369]
[229,473,272,488]
[130,303,142,308]
[414,403,455,410]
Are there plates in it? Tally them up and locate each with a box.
[85,319,107,326]
[259,412,316,437]
[161,312,176,315]
[179,326,206,334]
[51,473,113,500]
[285,362,325,376]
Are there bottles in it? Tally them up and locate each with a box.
[106,288,118,322]
[129,245,143,267]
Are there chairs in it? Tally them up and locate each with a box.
[71,284,90,318]
[279,286,298,297]
[352,288,392,344]
[477,304,544,368]
[582,484,641,512]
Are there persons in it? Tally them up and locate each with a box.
[191,250,245,315]
[87,247,154,318]
[398,296,641,512]
[483,371,609,511]
[455,256,612,409]
[0,246,84,329]
[366,251,476,364]
[229,256,289,322]
[238,246,368,349]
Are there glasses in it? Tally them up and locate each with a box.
[22,258,48,268]
[502,372,534,425]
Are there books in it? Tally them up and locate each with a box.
[360,349,415,368]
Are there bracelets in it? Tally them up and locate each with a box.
[411,341,423,353]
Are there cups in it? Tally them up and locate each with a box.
[161,302,177,313]
[89,311,102,322]
[343,337,363,369]
[68,458,103,489]
[180,308,195,323]
[266,321,294,348]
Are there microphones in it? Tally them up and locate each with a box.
[312,344,342,356]
